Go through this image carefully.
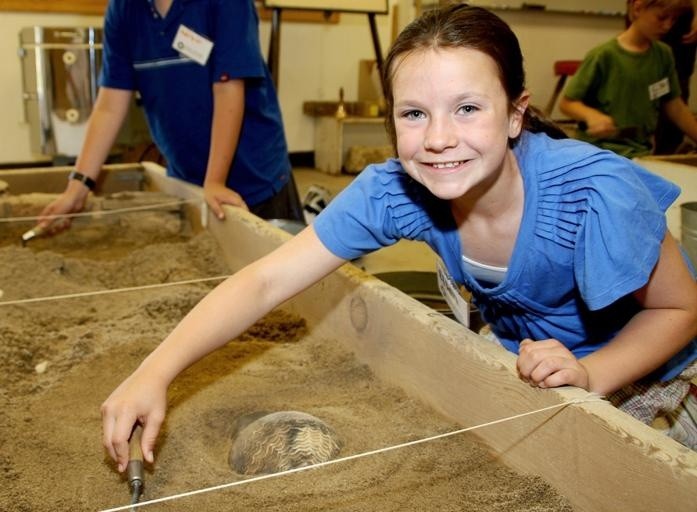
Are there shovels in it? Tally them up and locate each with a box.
[0,218,64,250]
[586,126,638,140]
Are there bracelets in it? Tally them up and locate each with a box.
[68,171,96,191]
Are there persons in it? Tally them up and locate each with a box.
[99,0,697,473]
[625,1,696,155]
[37,0,305,238]
[560,0,697,162]
